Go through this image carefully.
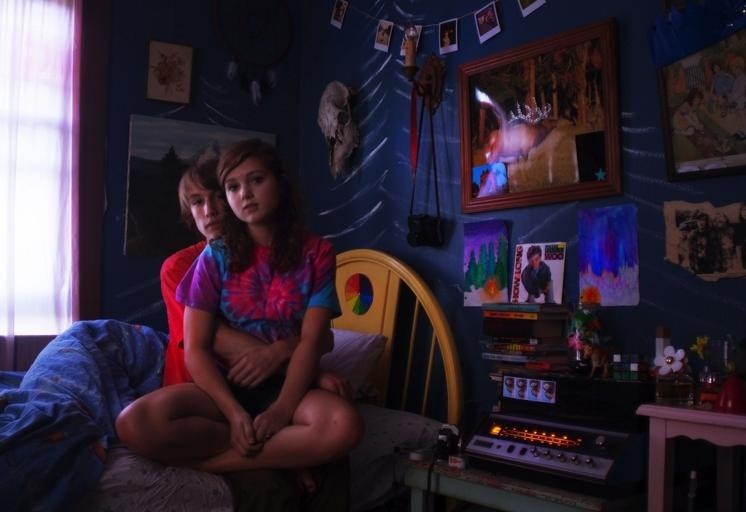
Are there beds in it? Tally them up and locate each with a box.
[0,248,461,511]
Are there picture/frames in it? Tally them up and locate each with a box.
[459,18,624,214]
[657,24,745,180]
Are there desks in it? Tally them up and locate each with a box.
[635,403,746,512]
[404,456,623,512]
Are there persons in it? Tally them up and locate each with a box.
[672,51,746,159]
[376,22,389,45]
[686,212,728,274]
[113,137,366,496]
[521,245,555,303]
[157,152,356,401]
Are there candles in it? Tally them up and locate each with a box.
[404,23,417,66]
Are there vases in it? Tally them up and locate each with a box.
[699,379,721,409]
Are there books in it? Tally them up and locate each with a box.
[479,303,570,372]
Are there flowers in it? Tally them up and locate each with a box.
[653,333,737,387]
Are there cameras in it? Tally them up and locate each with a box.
[407,213,443,248]
